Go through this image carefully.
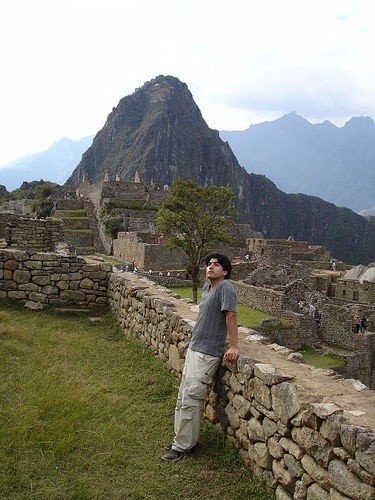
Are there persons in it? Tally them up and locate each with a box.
[309,303,316,318]
[161,253,239,461]
[118,251,122,258]
[148,268,152,275]
[244,254,250,261]
[158,270,163,276]
[166,271,171,276]
[176,272,180,277]
[120,264,129,272]
[132,258,138,272]
[331,261,336,271]
[68,244,76,254]
[355,317,367,333]
[298,301,304,307]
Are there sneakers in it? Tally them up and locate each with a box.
[166,441,174,450]
[162,448,186,461]
